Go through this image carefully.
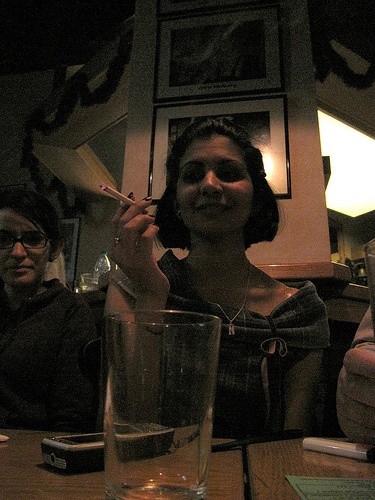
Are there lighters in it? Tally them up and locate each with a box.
[302,436,375,465]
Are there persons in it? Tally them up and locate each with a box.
[337,304,375,448]
[0,185,103,438]
[105,114,331,442]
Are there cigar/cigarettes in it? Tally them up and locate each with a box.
[102,184,150,217]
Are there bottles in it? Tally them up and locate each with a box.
[93,251,110,285]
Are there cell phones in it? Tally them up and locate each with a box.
[41,422,174,475]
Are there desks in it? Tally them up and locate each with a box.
[246,438,375,500]
[0,427,246,500]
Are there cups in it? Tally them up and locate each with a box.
[102,309,221,500]
[82,271,99,292]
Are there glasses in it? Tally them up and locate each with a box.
[0,229,49,249]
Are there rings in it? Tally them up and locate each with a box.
[114,237,122,244]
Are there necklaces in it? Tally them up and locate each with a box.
[188,260,252,335]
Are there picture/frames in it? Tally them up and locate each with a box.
[56,216,81,291]
[145,0,292,203]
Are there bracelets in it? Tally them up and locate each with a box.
[351,335,375,346]
[134,316,164,337]
